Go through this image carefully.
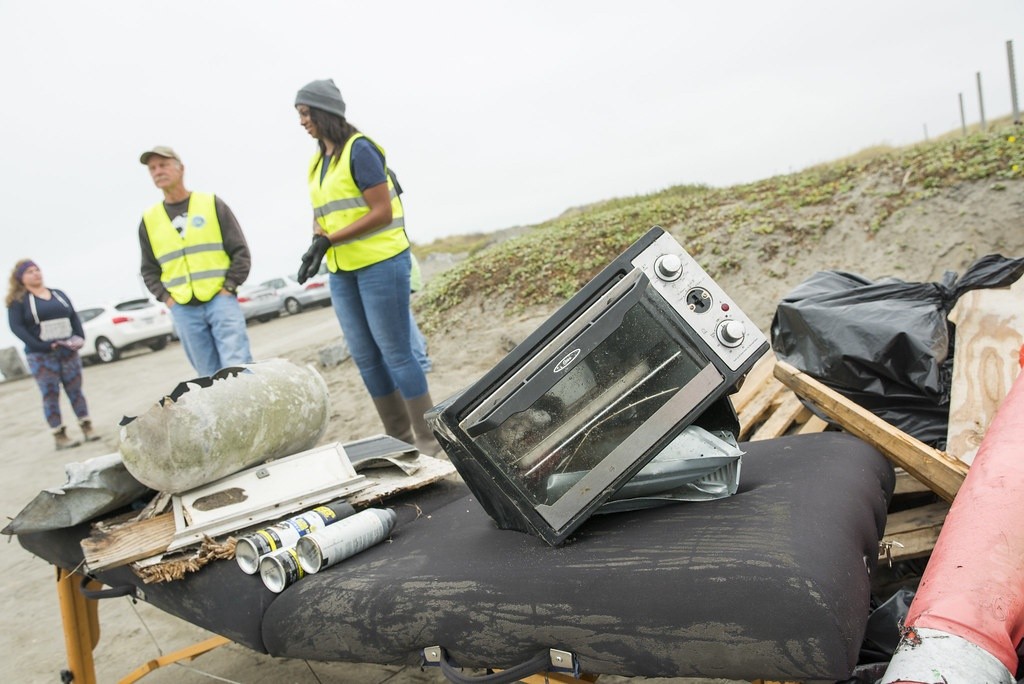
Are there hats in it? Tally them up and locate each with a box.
[295,79,346,117]
[140,146,179,164]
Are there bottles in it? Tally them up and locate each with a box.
[236,499,356,574]
[259,545,303,594]
[295,508,398,574]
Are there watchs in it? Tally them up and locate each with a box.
[225,285,235,292]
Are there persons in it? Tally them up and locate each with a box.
[4,259,101,450]
[407,251,433,373]
[138,145,254,378]
[295,79,443,457]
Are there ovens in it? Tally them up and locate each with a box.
[421,226,770,543]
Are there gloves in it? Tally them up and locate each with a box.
[297,234,333,284]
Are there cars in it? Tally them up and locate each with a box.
[262,270,333,315]
[66,296,175,362]
[153,279,281,342]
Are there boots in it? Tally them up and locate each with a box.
[80,419,101,442]
[51,425,81,450]
[373,387,413,445]
[405,392,449,460]
